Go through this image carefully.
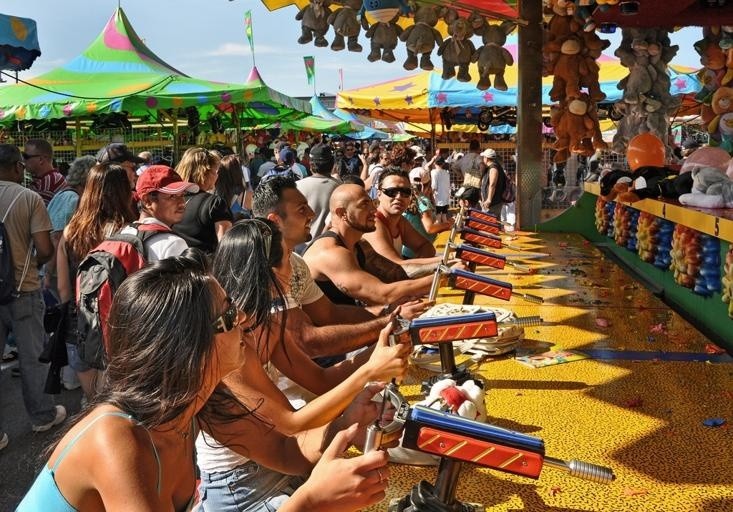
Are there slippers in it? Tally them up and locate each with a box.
[2,351,18,363]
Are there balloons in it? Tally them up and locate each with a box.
[626,133,666,172]
[679,147,730,176]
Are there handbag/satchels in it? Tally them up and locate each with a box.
[463,166,482,190]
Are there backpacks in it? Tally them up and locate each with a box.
[501,172,517,205]
[0,188,35,306]
[75,222,180,370]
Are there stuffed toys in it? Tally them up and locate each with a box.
[614,27,682,133]
[365,12,404,63]
[295,0,333,47]
[693,25,733,156]
[437,9,483,81]
[470,17,514,90]
[600,166,733,209]
[541,0,609,162]
[400,2,448,71]
[327,0,369,52]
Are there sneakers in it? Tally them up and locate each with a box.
[32,405,66,432]
[0,433,9,449]
[11,367,21,377]
[64,382,81,391]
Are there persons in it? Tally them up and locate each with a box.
[3,137,511,511]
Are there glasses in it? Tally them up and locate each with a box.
[346,148,353,151]
[121,165,137,171]
[404,160,415,165]
[209,296,238,335]
[379,185,411,198]
[336,154,343,157]
[17,161,26,169]
[21,150,40,160]
[203,147,209,156]
[236,218,272,262]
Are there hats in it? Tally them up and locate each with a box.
[275,142,290,150]
[408,166,430,186]
[436,155,445,162]
[95,143,145,163]
[246,143,260,154]
[309,142,332,161]
[279,149,294,162]
[455,185,479,202]
[136,165,200,200]
[255,161,277,178]
[480,149,497,159]
[412,147,425,160]
